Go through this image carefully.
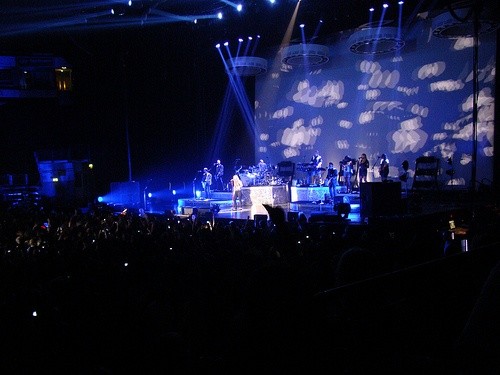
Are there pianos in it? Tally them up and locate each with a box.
[296,163,326,187]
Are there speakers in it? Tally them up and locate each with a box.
[361,181,401,216]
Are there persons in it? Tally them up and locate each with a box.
[310,150,323,185]
[326,162,338,199]
[201,167,212,199]
[0,192,500,375]
[215,159,226,192]
[379,154,390,183]
[342,155,353,189]
[231,175,243,211]
[257,159,268,172]
[357,154,369,187]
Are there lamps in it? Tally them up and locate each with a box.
[55,68,72,92]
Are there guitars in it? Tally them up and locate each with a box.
[325,176,333,187]
[202,174,210,188]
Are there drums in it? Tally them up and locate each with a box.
[239,167,271,186]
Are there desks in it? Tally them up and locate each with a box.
[242,185,288,206]
[291,185,347,202]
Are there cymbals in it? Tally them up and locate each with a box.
[250,167,259,169]
[243,170,250,172]
[267,163,276,165]
